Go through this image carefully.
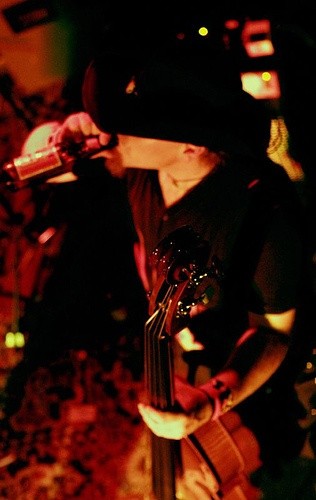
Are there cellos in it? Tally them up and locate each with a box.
[121,224,272,499]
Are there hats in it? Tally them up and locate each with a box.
[82,37,246,150]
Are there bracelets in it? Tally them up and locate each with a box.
[206,377,233,414]
[198,385,221,421]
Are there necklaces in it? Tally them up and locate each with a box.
[164,169,204,189]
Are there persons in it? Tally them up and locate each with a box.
[2,26,315,492]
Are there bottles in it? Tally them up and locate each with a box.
[2,132,119,193]
